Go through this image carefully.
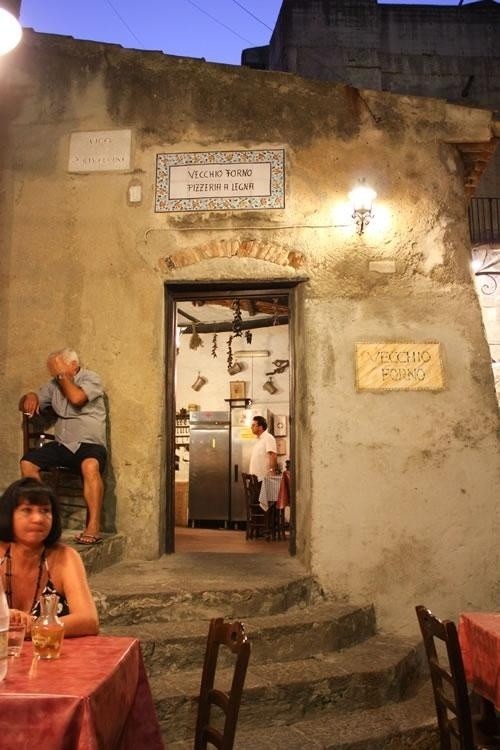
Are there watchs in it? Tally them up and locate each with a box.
[57,374,64,380]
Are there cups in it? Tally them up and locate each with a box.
[191,376,206,391]
[263,381,277,395]
[9,626,25,659]
[227,362,241,376]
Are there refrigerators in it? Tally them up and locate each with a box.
[230,408,272,530]
[188,410,231,530]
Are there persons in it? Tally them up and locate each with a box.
[19,347,108,545]
[247,416,277,538]
[0,475,100,639]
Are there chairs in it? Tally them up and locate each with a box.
[22,412,107,531]
[415,605,500,750]
[193,617,250,750]
[242,473,276,541]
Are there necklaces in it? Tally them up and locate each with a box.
[5,547,46,611]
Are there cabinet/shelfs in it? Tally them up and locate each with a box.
[224,398,251,409]
[175,408,189,450]
[175,482,189,526]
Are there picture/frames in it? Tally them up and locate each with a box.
[155,149,285,213]
[230,381,246,399]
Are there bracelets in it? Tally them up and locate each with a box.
[270,469,275,471]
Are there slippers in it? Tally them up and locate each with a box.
[73,533,103,544]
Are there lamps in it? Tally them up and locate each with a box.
[234,351,270,357]
[346,176,377,236]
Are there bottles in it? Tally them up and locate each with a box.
[36,434,47,450]
[31,594,64,659]
[0,580,10,681]
